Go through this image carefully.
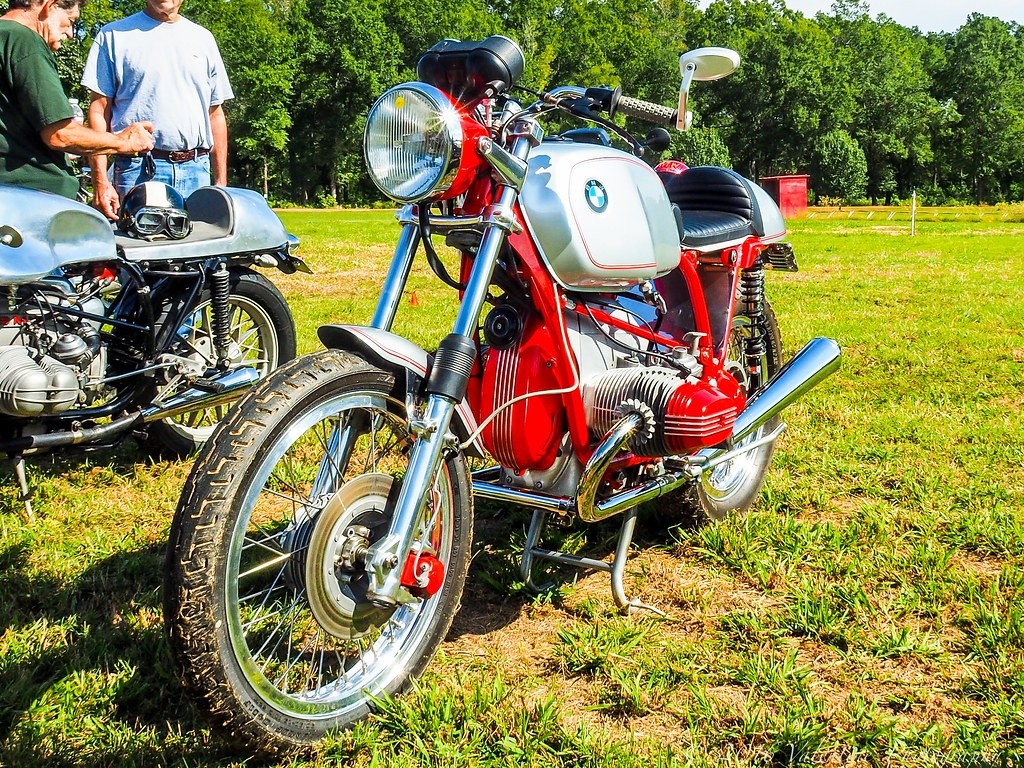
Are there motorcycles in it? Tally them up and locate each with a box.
[0,184,314,523]
[165,34,842,764]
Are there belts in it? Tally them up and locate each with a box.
[141,146,208,163]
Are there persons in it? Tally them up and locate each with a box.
[0,0,154,201]
[80,0,237,422]
